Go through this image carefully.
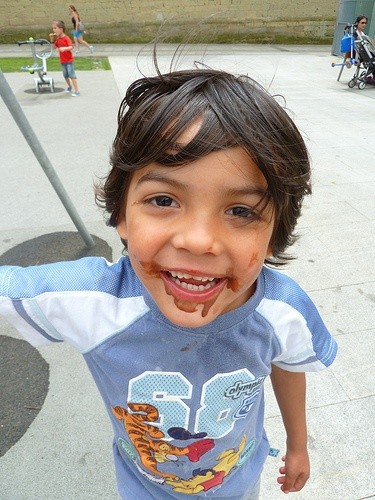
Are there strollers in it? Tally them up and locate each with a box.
[343,24,375,90]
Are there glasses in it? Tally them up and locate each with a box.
[361,22,367,24]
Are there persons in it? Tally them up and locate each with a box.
[0,12,338,499]
[69,4,94,55]
[49,19,82,96]
[354,15,375,79]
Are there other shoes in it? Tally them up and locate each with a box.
[66,87,72,93]
[74,51,80,54]
[89,46,94,54]
[72,91,80,97]
